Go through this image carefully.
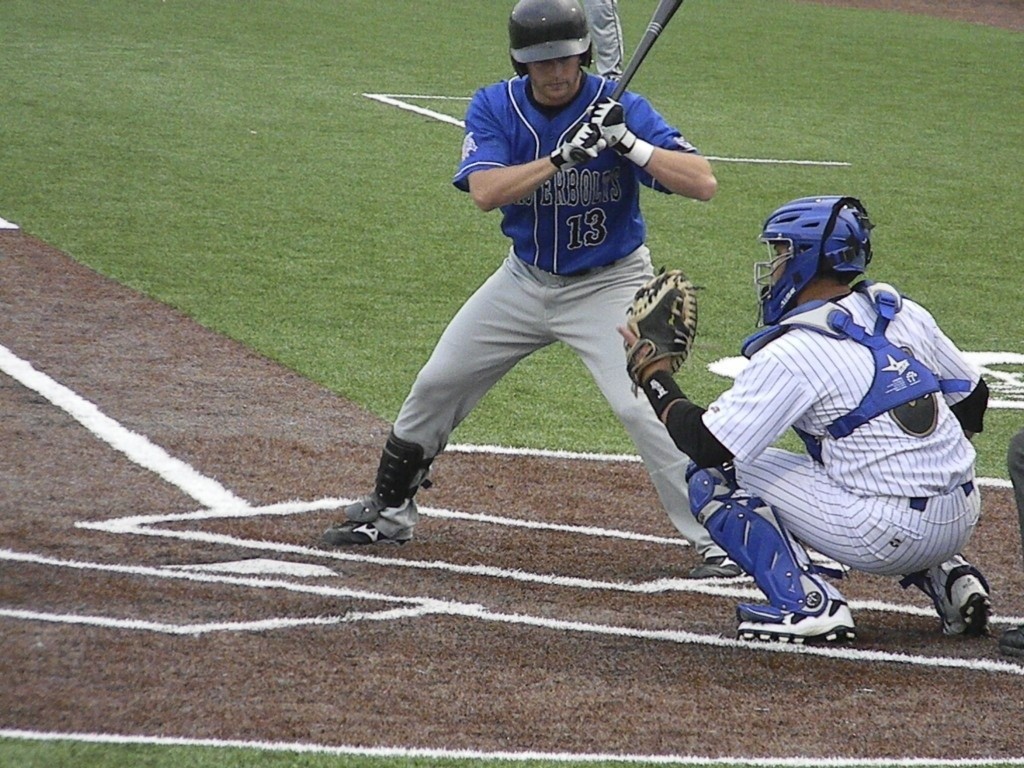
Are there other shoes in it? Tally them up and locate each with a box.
[999,624,1024,657]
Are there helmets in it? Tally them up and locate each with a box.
[752,195,875,327]
[508,0,592,79]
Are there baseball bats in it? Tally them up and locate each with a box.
[570,0,683,165]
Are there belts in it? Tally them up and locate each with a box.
[910,481,975,512]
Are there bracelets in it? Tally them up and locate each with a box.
[643,369,685,422]
[624,138,654,168]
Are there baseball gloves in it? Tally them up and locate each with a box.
[624,269,698,388]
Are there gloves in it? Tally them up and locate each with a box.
[550,120,607,174]
[586,95,655,167]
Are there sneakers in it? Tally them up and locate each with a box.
[689,554,755,585]
[940,574,995,638]
[323,519,411,545]
[737,600,856,648]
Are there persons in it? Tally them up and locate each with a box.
[561,0,624,82]
[999,434,1023,656]
[615,195,993,645]
[321,0,754,586]
